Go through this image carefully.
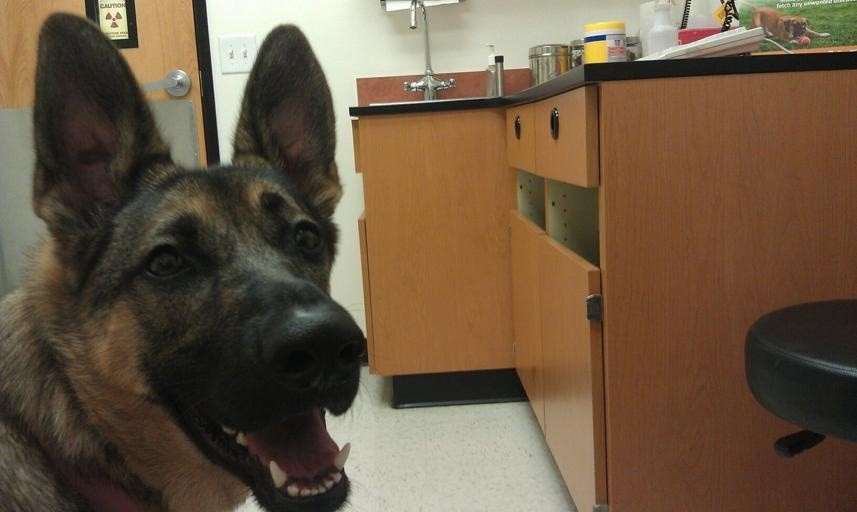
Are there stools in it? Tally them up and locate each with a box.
[746,300,855,455]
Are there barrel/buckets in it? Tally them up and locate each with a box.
[584,21,626,63]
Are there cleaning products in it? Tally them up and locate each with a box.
[484,44,497,95]
[646,0,678,57]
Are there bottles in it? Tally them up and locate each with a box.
[528,1,677,86]
[495,56,504,97]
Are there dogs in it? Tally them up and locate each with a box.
[739,0,831,47]
[0,9,368,512]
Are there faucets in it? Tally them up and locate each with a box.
[403,0,456,101]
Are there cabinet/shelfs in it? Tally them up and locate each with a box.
[345,97,514,378]
[505,54,857,512]
